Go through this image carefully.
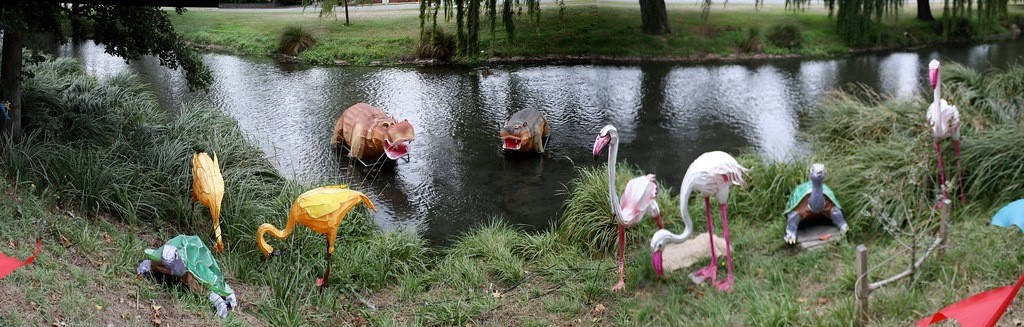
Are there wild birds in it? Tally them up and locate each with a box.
[926,60,967,208]
[592,125,755,295]
[191,151,378,296]
